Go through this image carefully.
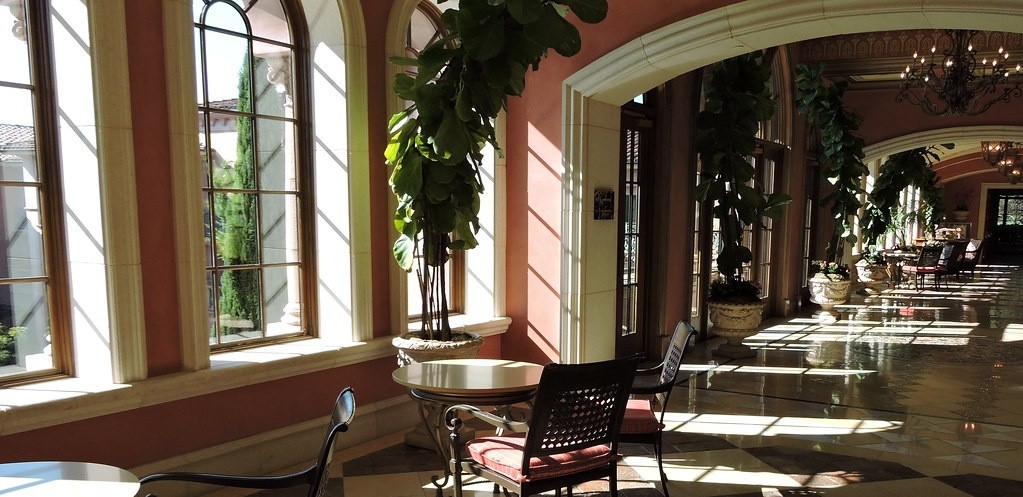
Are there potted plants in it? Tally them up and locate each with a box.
[690,46,791,355]
[793,60,870,309]
[855,141,950,293]
[381,0,607,442]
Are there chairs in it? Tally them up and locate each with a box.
[444,349,645,497]
[133,396,354,497]
[554,318,694,497]
[899,235,984,290]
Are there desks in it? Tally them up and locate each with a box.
[391,359,543,497]
[0,460,140,497]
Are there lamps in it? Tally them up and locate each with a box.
[893,27,1023,117]
[982,140,1022,185]
